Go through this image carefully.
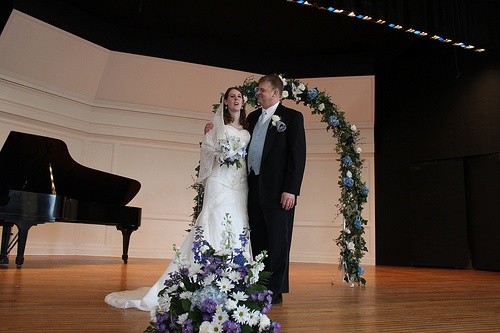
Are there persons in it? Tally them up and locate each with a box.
[204,75,306,304]
[103,87,253,311]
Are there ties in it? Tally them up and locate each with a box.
[259,110,267,122]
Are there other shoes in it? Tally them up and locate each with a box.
[271,292,282,302]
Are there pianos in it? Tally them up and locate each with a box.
[0,130,142,264]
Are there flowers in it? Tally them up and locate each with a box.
[144,211,282,333]
[215,134,248,168]
[271,115,286,133]
[186,74,370,286]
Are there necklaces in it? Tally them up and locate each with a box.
[230,122,243,130]
